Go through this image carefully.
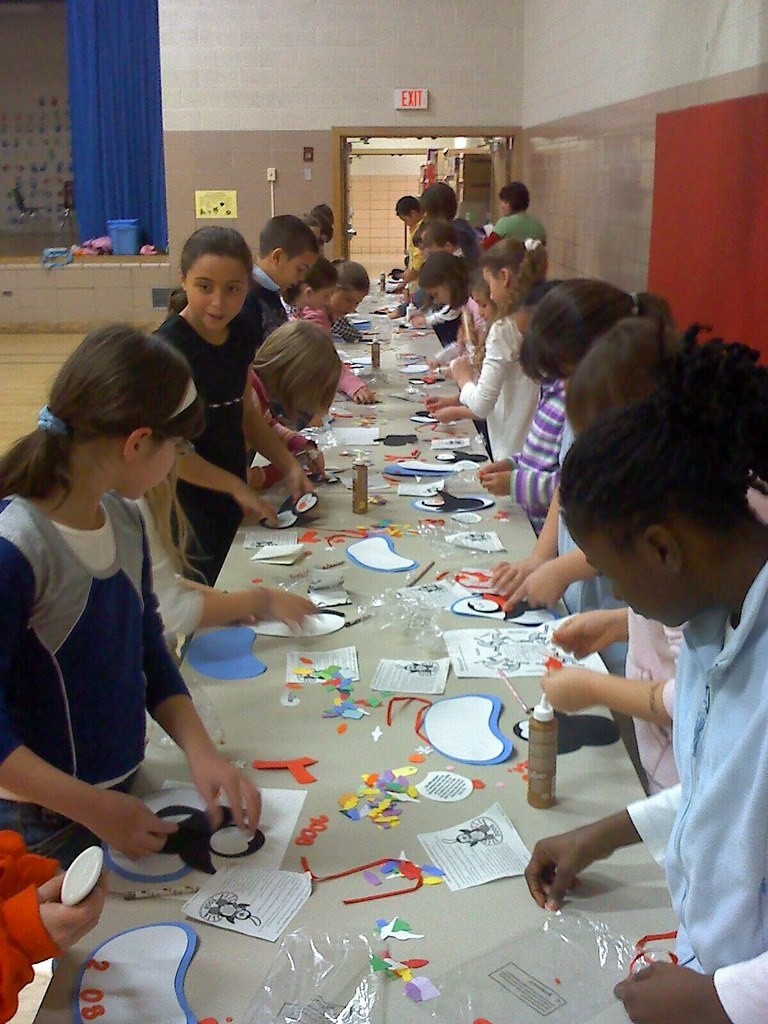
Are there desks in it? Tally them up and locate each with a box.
[31,280,680,1024]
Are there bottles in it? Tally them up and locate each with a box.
[352,450,368,514]
[528,693,558,808]
[381,272,385,291]
[372,339,380,368]
[297,449,320,464]
[406,303,417,321]
[461,311,479,354]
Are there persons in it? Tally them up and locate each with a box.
[129,184,768,1024]
[0,324,261,860]
[0,828,105,1024]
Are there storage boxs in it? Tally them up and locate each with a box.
[107,219,143,255]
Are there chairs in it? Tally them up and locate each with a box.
[13,181,75,241]
